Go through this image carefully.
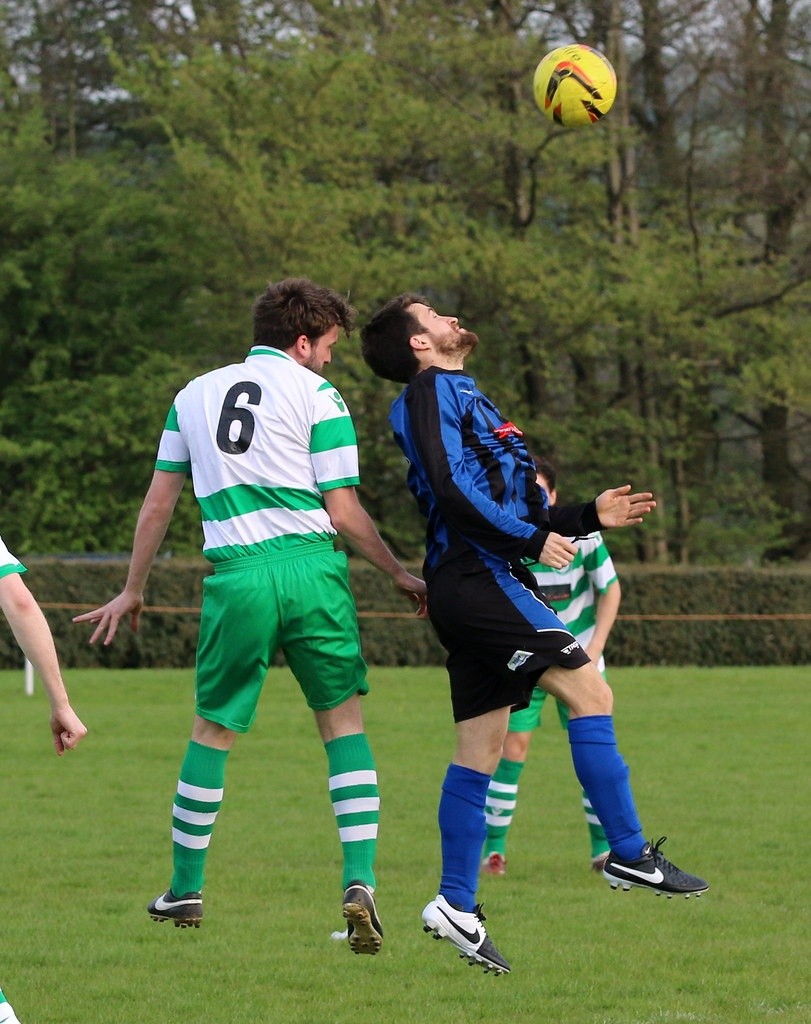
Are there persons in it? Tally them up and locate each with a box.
[360,292,710,974]
[0,534,90,758]
[66,276,428,955]
[478,465,623,873]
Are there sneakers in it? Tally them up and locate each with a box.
[146,888,202,928]
[420,894,511,977]
[602,836,710,899]
[592,850,610,874]
[480,851,506,875]
[341,879,383,955]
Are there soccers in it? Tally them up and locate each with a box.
[532,43,620,129]
